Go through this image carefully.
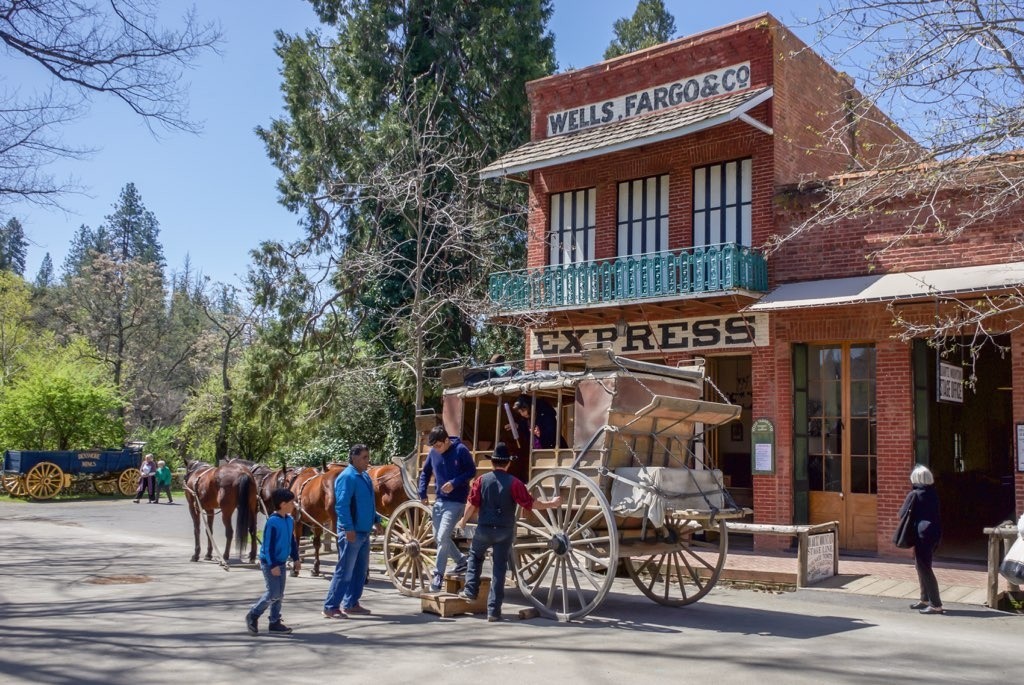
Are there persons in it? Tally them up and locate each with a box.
[417,426,476,593]
[456,444,562,622]
[897,466,942,615]
[245,490,300,634]
[504,393,569,450]
[322,444,377,619]
[150,461,173,505]
[132,454,156,504]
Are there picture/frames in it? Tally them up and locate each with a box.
[731,423,743,442]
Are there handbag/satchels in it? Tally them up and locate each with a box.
[893,489,919,547]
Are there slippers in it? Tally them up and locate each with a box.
[919,605,943,614]
[910,600,928,609]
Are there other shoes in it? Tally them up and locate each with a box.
[488,614,498,622]
[133,501,140,503]
[147,501,151,504]
[342,602,371,615]
[429,574,443,593]
[245,613,259,635]
[322,608,347,618]
[457,591,476,600]
[168,501,173,503]
[269,620,292,633]
[449,559,469,573]
[153,500,158,504]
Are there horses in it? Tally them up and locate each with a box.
[226,455,309,577]
[283,455,423,585]
[183,456,259,567]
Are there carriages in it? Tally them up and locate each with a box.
[181,339,743,618]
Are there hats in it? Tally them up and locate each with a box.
[484,443,517,461]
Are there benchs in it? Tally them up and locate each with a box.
[585,519,839,587]
